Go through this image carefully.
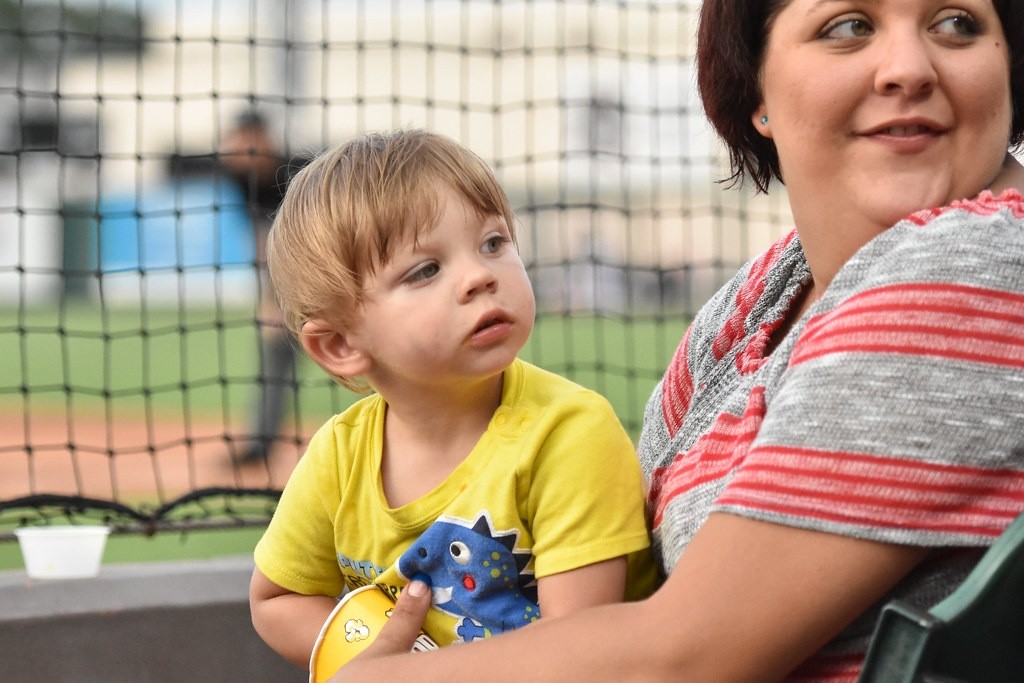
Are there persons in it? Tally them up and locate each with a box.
[211,114,315,472]
[249,128,652,668]
[324,0,1024,683]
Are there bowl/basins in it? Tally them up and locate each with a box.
[13,525,112,579]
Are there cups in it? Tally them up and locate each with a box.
[308,585,440,683]
